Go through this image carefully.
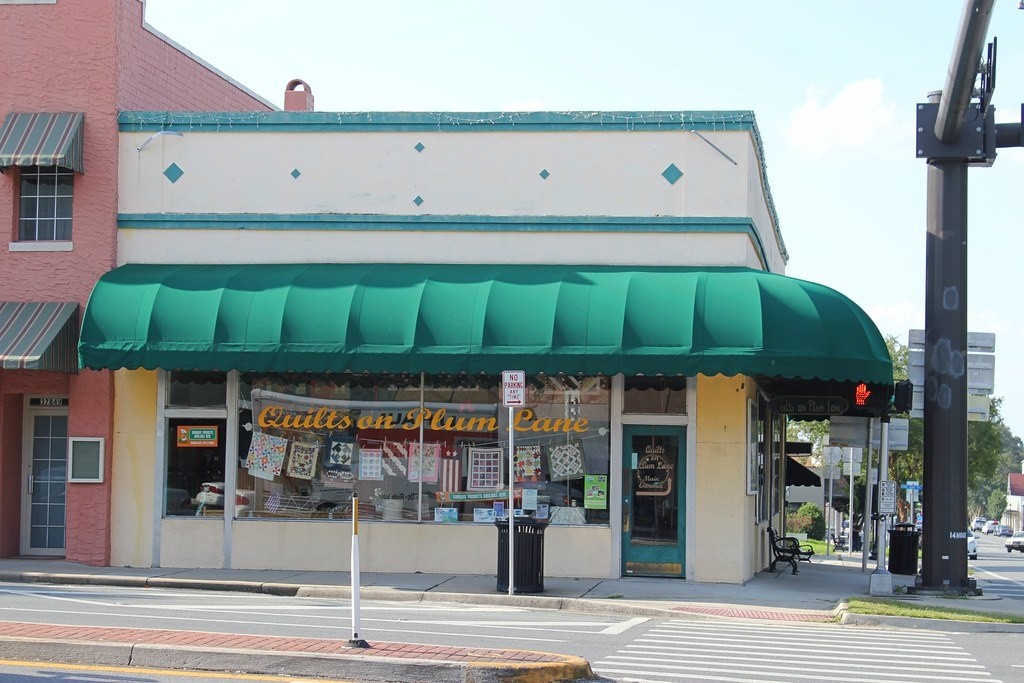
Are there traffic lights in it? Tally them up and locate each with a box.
[851,383,890,413]
[897,381,914,411]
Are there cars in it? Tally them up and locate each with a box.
[165,487,194,517]
[1005,531,1024,553]
[993,525,1014,537]
[503,457,584,523]
[197,482,225,507]
[310,456,496,522]
[967,527,980,560]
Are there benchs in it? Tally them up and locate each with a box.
[792,537,815,563]
[832,534,845,552]
[767,527,800,575]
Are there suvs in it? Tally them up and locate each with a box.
[984,521,999,534]
[31,465,67,532]
[973,520,986,531]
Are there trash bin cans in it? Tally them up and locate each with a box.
[494,517,549,593]
[886,521,922,575]
